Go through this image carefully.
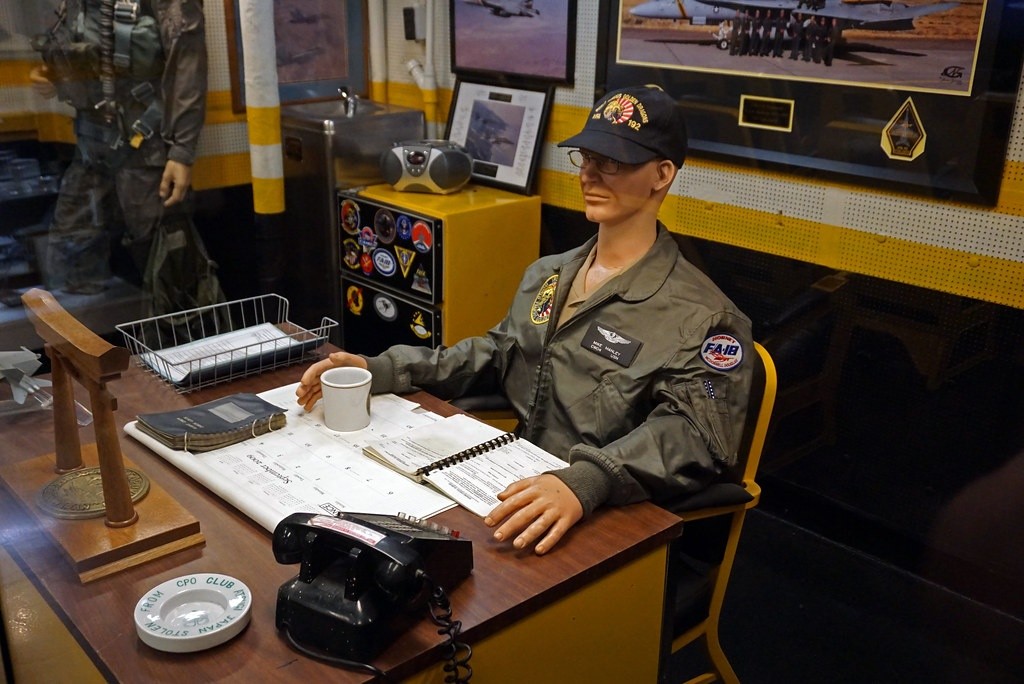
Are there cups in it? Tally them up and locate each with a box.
[320,367,372,431]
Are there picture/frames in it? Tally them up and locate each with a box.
[226,0,372,114]
[443,77,555,195]
[596,1,1024,206]
[448,0,578,88]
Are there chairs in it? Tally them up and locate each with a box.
[453,338,778,683]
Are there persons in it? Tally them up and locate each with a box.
[729,8,840,66]
[30,1,206,292]
[296,86,755,556]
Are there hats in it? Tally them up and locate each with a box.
[557,83,688,169]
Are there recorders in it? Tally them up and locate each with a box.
[380,136,475,195]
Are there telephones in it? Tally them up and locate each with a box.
[272,511,474,665]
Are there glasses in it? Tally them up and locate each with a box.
[566,149,620,175]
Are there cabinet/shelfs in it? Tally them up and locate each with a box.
[0,322,682,684]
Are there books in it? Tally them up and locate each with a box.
[136,391,290,452]
[362,414,569,519]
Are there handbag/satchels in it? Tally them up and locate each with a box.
[140,190,220,344]
